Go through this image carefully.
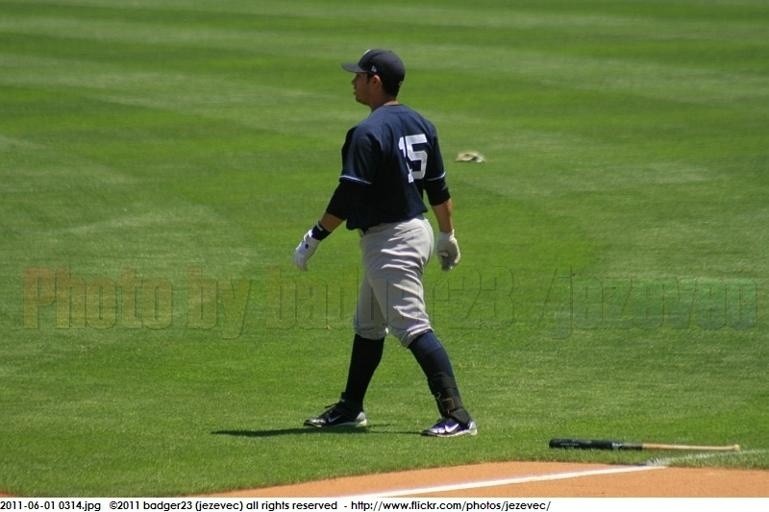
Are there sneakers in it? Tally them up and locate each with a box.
[303,391,367,429]
[421,413,477,438]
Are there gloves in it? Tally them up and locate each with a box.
[291,229,321,272]
[435,228,461,272]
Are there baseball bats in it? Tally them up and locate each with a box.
[549,439,740,452]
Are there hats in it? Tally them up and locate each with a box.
[341,47,406,87]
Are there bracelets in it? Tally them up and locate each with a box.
[311,221,332,242]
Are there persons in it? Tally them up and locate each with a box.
[290,47,480,441]
